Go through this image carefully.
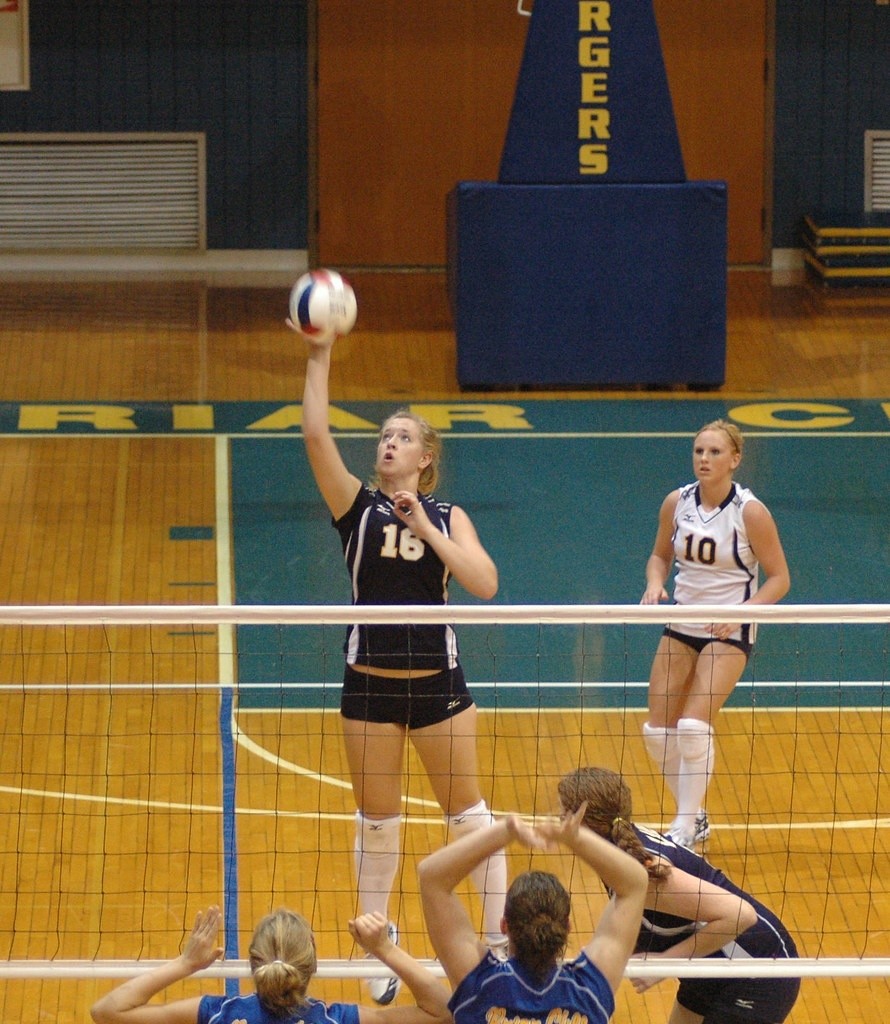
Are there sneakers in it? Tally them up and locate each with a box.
[363,921,401,1004]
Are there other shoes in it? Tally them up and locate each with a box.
[694,810,710,842]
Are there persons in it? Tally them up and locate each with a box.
[88,903,452,1023]
[283,319,508,1006]
[638,419,790,847]
[558,767,802,1024]
[418,800,648,1024]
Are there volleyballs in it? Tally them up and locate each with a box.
[290,266,358,340]
